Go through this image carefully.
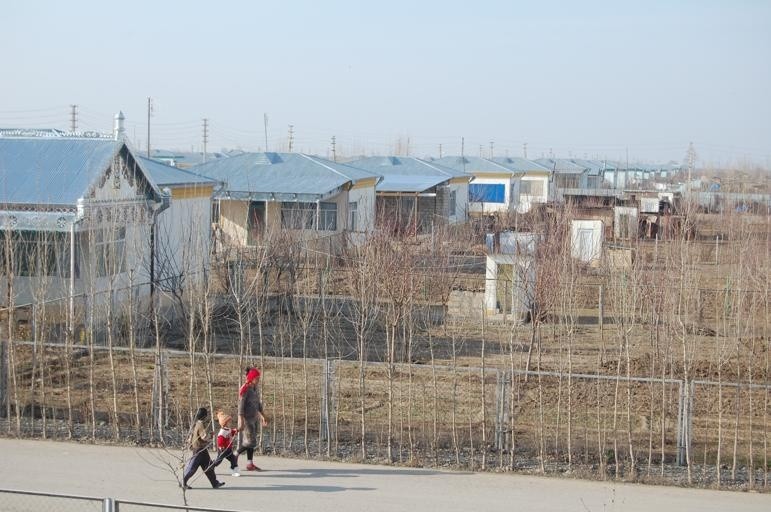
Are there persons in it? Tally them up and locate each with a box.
[212,410,244,477]
[233,368,270,472]
[180,407,225,490]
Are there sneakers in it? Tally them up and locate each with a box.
[247,464,262,471]
[179,481,193,489]
[230,468,241,478]
[212,481,226,490]
[233,452,239,463]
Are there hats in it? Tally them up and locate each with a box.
[218,412,233,427]
